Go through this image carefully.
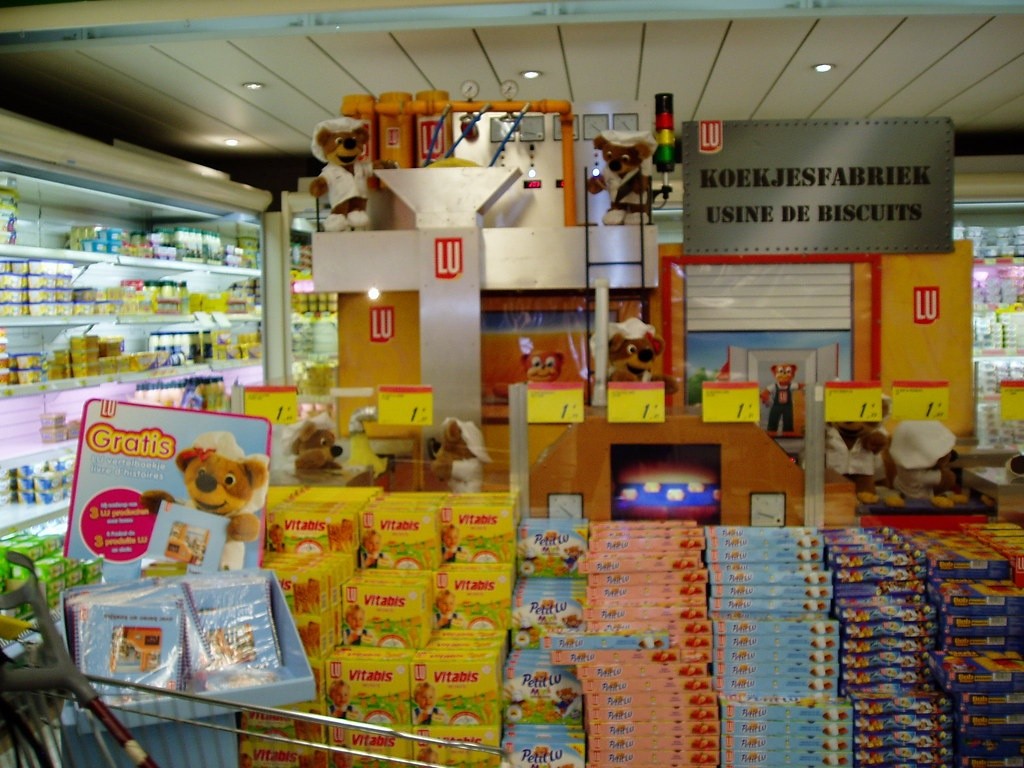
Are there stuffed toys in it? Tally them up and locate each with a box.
[587,317,666,380]
[761,364,971,511]
[432,418,492,491]
[307,119,385,231]
[144,429,269,571]
[522,350,564,383]
[284,421,339,485]
[588,128,659,229]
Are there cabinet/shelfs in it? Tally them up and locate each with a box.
[953,197,1024,401]
[264,189,338,388]
[0,109,272,535]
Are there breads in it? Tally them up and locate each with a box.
[856,492,968,508]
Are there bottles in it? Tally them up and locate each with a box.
[131,225,223,265]
[147,330,212,367]
[135,375,228,412]
[121,279,190,313]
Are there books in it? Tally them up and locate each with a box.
[67,575,282,709]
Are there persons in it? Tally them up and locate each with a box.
[237,523,468,768]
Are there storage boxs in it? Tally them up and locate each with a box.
[0,486,1024,767]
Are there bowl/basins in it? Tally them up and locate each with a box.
[0,186,122,316]
[8,334,130,382]
[1,412,81,506]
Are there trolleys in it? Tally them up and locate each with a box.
[1,549,512,768]
